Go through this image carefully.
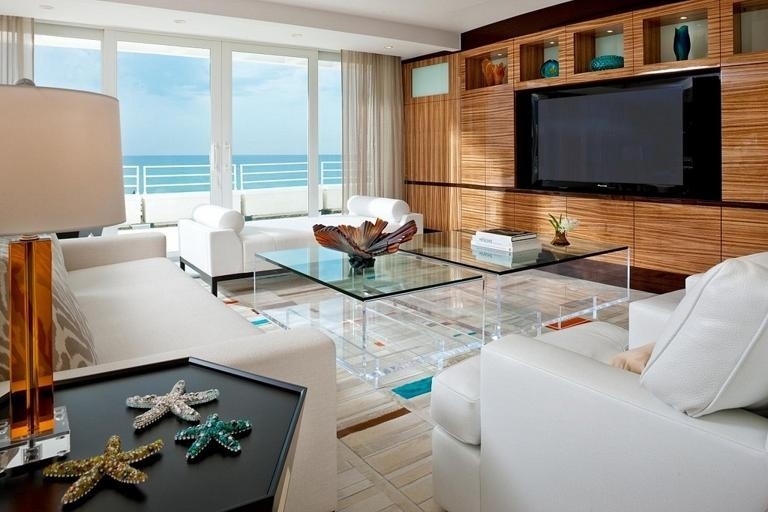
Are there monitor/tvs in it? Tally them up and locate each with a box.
[530,74,696,201]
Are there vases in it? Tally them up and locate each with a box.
[551,233,570,247]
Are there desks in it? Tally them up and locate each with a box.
[0,351,310,511]
[253,247,484,390]
[363,230,630,343]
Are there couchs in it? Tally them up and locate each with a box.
[0,231,337,512]
[430,251,768,512]
[178,195,423,297]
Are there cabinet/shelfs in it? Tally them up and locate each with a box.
[721,63,768,206]
[513,195,567,244]
[402,99,460,184]
[405,187,513,248]
[721,207,768,263]
[566,199,634,266]
[457,0,768,92]
[634,202,721,275]
[460,93,515,186]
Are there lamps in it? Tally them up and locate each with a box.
[0,87,126,479]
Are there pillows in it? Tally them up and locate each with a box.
[609,343,656,375]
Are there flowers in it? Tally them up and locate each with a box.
[547,213,578,233]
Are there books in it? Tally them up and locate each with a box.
[470,228,542,268]
